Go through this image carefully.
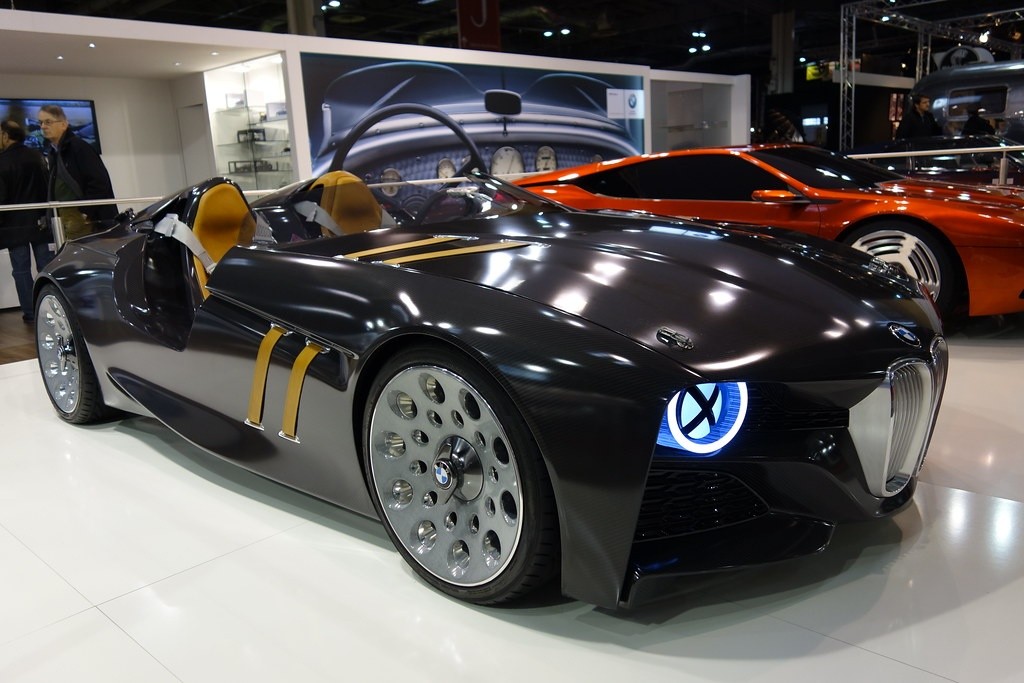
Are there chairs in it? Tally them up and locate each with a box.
[305,170,387,237]
[183,176,262,301]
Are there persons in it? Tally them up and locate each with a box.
[962,107,993,136]
[0,103,118,324]
[900,94,940,145]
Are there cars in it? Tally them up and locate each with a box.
[880,136,1024,190]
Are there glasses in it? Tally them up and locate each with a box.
[37,118,64,126]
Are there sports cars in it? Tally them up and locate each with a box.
[494,143,1024,337]
[31,102,952,613]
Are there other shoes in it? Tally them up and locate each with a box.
[22,313,37,325]
